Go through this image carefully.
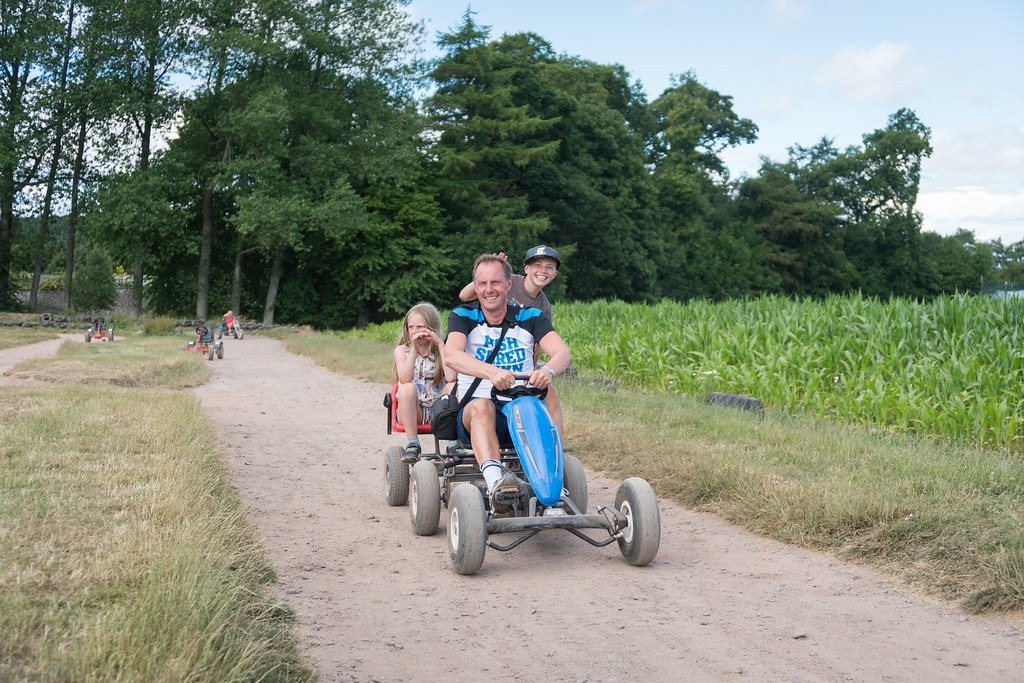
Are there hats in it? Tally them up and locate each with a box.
[523,245,561,269]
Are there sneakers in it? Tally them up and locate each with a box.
[554,487,569,507]
[401,443,421,461]
[490,472,518,518]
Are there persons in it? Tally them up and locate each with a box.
[391,303,458,463]
[458,244,562,369]
[223,311,236,333]
[443,254,572,514]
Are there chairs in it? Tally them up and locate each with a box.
[196,326,214,343]
[392,383,433,433]
[95,322,106,331]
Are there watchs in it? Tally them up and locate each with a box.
[541,365,554,377]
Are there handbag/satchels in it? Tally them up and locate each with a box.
[431,394,458,441]
